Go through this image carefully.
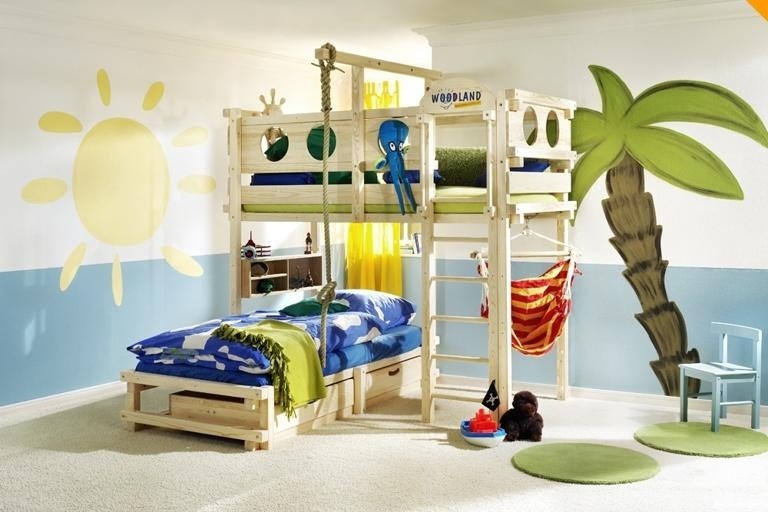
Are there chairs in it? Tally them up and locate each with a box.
[678,321,762,432]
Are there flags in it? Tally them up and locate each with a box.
[481,381,501,413]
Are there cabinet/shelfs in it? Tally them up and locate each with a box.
[241,253,323,299]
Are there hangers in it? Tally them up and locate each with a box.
[470,214,583,259]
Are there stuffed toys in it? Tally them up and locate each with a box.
[499,390,544,442]
[376,120,417,217]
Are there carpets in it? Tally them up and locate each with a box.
[633,421,768,458]
[513,442,661,485]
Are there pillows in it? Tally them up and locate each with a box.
[279,289,417,330]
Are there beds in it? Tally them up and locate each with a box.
[223,45,578,428]
[119,324,440,451]
